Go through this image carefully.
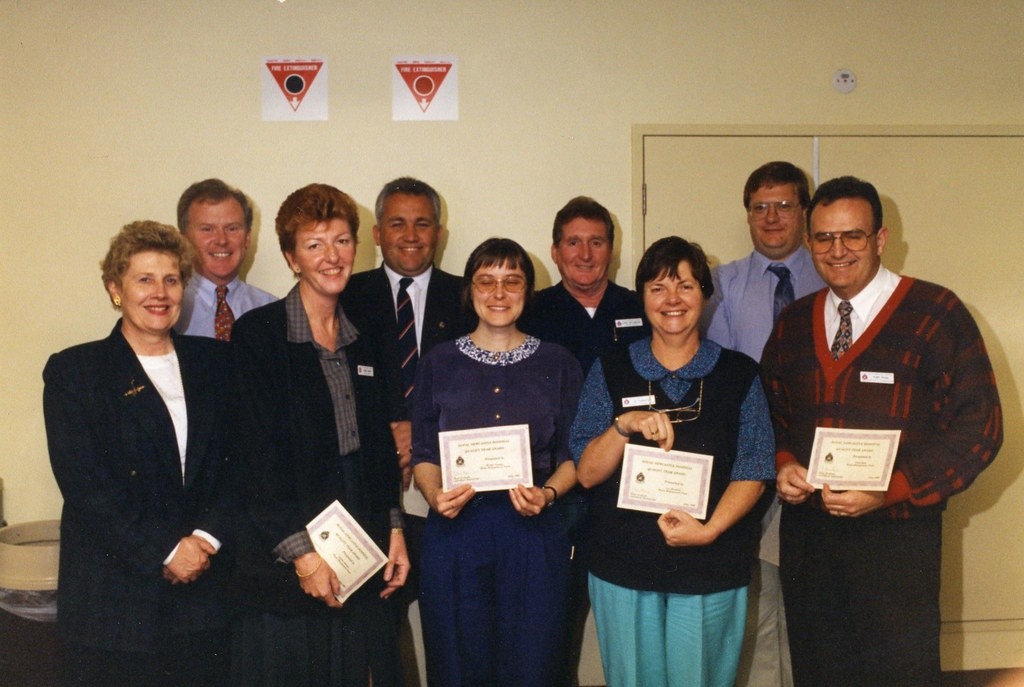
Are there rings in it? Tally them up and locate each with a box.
[652,429,658,435]
[397,449,399,455]
[408,447,414,455]
[838,510,841,518]
[451,501,457,508]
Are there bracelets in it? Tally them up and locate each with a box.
[296,559,321,577]
[613,418,631,437]
[391,528,403,534]
[543,486,557,501]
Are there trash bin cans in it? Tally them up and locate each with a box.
[0,519,61,687]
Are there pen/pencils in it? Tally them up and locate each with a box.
[612,328,617,342]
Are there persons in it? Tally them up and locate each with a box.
[700,160,829,687]
[41,177,478,687]
[759,175,1003,687]
[411,238,586,687]
[527,193,653,383]
[568,236,777,687]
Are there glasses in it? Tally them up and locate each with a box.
[646,398,701,424]
[807,230,877,254]
[746,200,801,218]
[471,275,527,294]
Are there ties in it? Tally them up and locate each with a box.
[214,286,234,341]
[767,264,795,324]
[831,301,854,360]
[396,278,419,402]
